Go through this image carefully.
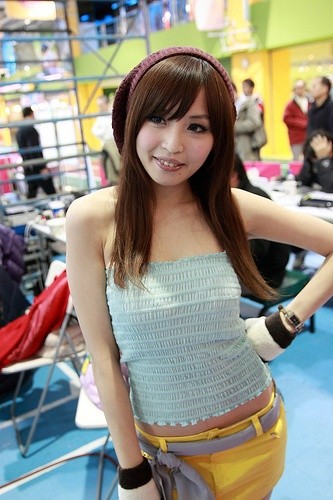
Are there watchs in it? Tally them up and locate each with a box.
[278,305,306,333]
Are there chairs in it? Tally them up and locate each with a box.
[0,260,82,457]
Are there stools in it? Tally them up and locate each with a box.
[231,270,315,336]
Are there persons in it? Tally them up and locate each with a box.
[0,70,332,405]
[65,47,333,500]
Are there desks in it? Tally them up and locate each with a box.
[243,181,333,269]
[32,218,76,323]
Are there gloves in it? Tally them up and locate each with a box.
[118,456,160,500]
[244,310,297,363]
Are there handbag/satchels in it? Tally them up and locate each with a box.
[251,128,267,150]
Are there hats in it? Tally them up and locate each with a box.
[112,47,237,156]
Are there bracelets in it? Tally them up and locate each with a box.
[265,312,298,349]
[117,457,153,490]
